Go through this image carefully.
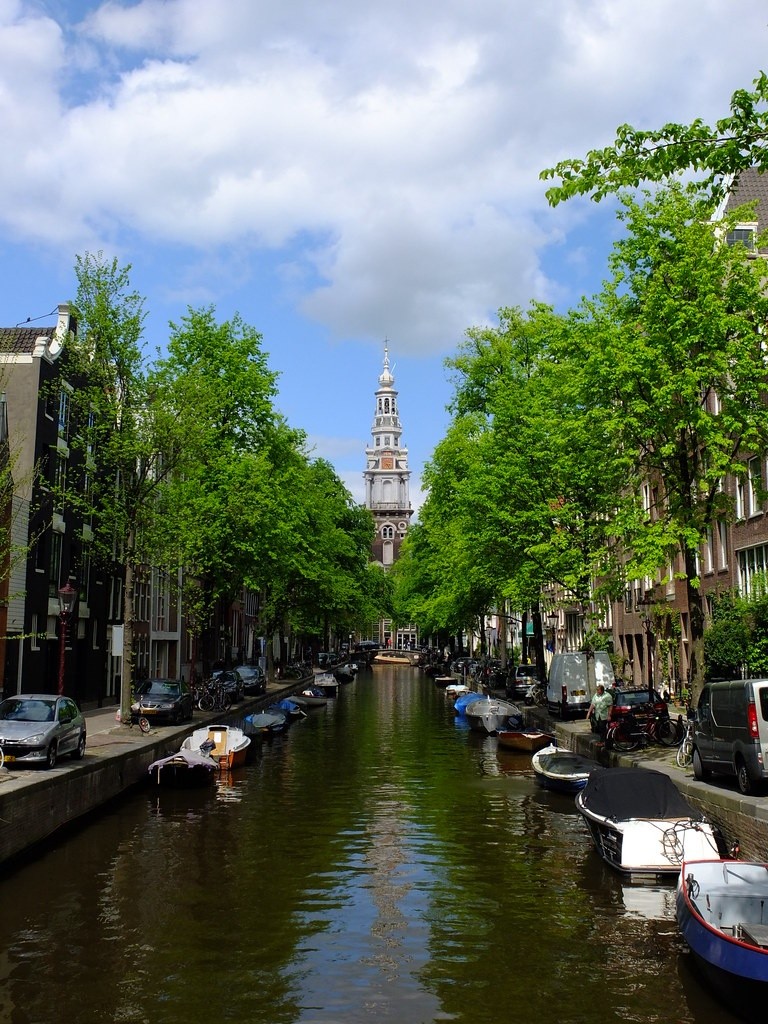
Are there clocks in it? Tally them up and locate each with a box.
[383,458,392,469]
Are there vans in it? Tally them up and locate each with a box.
[546,651,616,717]
[685,678,768,793]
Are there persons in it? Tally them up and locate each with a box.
[404,637,408,650]
[388,638,391,649]
[586,685,613,747]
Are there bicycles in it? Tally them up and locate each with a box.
[191,680,232,712]
[474,668,485,684]
[535,687,547,708]
[275,660,314,680]
[131,707,151,732]
[607,705,692,768]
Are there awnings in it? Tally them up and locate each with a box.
[518,623,546,638]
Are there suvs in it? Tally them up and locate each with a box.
[0,693,87,769]
[524,680,542,705]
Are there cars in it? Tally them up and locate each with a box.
[131,679,194,724]
[354,641,382,650]
[207,666,267,704]
[450,657,537,696]
[589,685,671,737]
[319,649,349,668]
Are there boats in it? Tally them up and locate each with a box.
[148,708,286,794]
[270,660,369,716]
[530,741,768,991]
[437,675,554,752]
